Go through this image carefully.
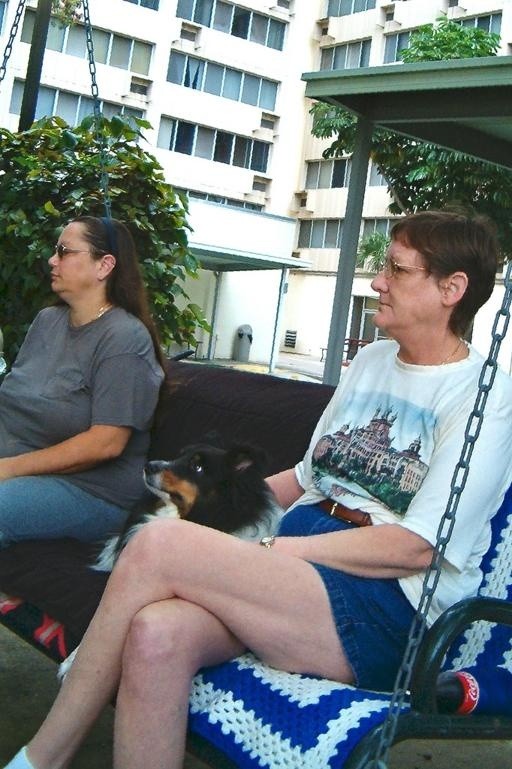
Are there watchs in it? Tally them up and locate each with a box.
[259,535,276,549]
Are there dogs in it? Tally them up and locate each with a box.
[87,440,286,572]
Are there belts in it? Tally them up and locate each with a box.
[318,498,372,527]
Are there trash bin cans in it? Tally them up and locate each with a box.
[232,326,253,363]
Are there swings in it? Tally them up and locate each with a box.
[0,0,512,764]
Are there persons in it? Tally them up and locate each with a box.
[1,216,512,769]
[1,217,178,544]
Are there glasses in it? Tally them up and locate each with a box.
[54,245,90,257]
[379,259,430,279]
